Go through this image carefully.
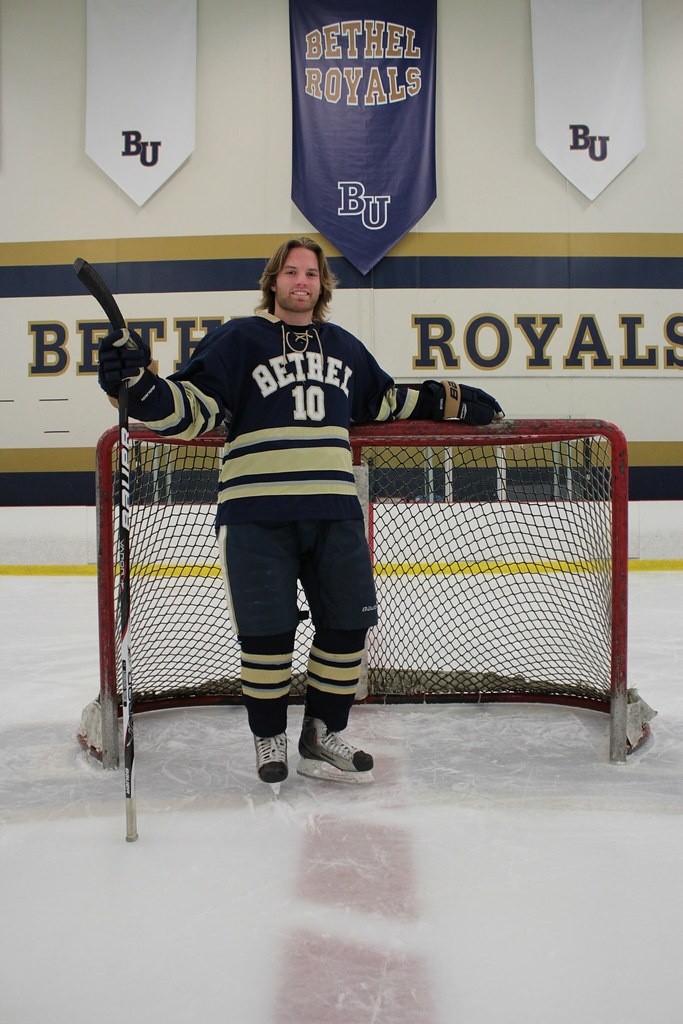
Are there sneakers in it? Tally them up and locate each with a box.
[254,732,291,800]
[297,718,374,783]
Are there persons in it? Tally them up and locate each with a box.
[96,237,506,793]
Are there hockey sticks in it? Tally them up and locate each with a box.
[74,256,142,845]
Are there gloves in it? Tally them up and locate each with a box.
[421,380,506,426]
[98,327,151,399]
